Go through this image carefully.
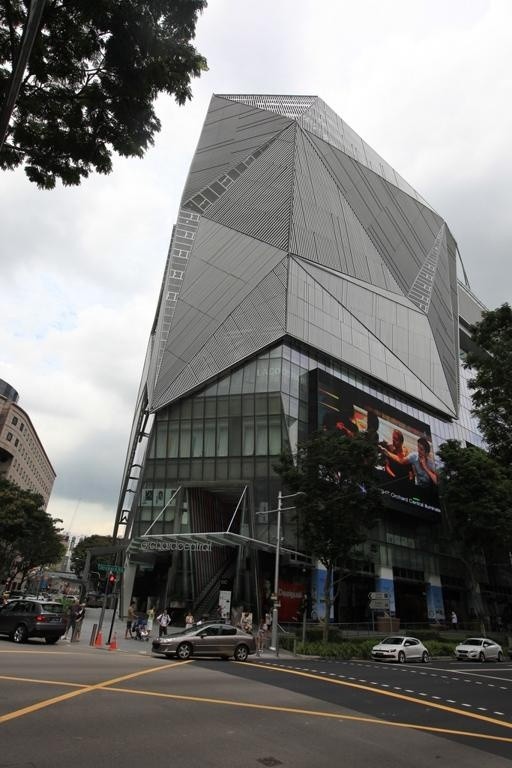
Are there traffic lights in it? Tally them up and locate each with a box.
[106,573,118,583]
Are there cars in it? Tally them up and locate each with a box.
[371,635,432,665]
[7,592,80,603]
[150,621,257,662]
[450,636,505,663]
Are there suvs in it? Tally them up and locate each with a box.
[0,599,68,646]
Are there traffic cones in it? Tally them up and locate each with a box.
[109,632,118,651]
[94,631,105,649]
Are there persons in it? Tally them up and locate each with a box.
[146,604,155,639]
[377,436,439,506]
[260,579,274,617]
[226,611,272,657]
[339,411,382,446]
[321,397,360,440]
[140,625,148,635]
[125,600,139,638]
[64,599,84,642]
[195,616,207,625]
[450,609,457,630]
[378,429,416,497]
[74,603,87,641]
[2,594,10,606]
[185,609,194,629]
[156,609,172,636]
[47,595,55,601]
[382,611,389,617]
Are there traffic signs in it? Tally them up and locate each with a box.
[367,592,393,599]
[369,599,391,609]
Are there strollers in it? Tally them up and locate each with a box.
[132,616,150,642]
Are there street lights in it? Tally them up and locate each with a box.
[254,490,308,653]
